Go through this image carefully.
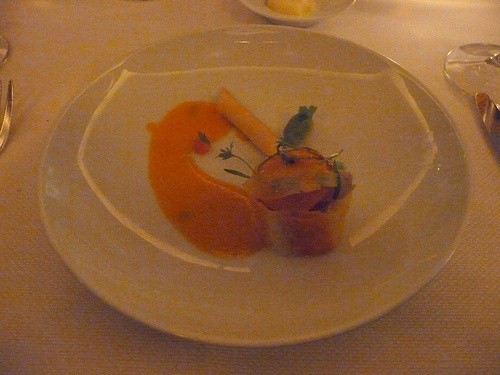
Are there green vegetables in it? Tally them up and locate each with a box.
[198,103,343,201]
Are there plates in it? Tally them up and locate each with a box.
[36,24,476,348]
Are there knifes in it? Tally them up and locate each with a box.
[475,92,500,162]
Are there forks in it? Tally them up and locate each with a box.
[0,79,15,156]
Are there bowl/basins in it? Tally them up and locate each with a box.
[238,0,356,27]
